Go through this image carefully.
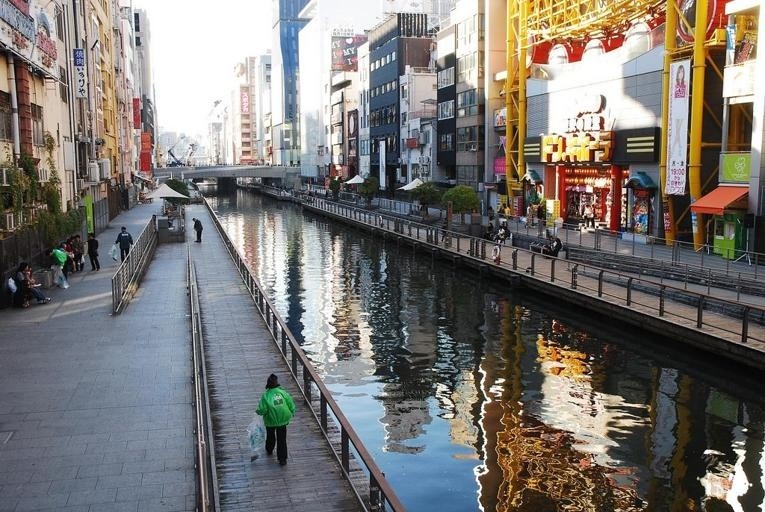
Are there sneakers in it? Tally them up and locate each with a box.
[38,297,51,303]
[267,451,287,465]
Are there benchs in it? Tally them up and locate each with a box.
[479,225,513,245]
[530,238,563,254]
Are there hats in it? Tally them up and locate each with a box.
[265,374,280,389]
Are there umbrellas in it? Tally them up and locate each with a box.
[344,174,364,192]
[396,178,424,191]
[145,184,189,214]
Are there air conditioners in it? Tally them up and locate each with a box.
[88,158,111,182]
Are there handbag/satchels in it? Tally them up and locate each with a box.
[8,278,17,293]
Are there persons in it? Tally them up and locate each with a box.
[16,235,84,308]
[115,226,134,262]
[344,183,352,192]
[86,233,100,271]
[584,204,595,228]
[488,203,562,257]
[255,373,295,464]
[192,218,203,242]
